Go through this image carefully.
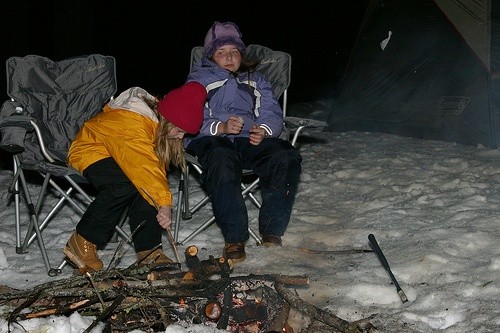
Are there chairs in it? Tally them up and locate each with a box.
[0,54,135,277]
[174,45,328,245]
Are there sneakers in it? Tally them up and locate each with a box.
[262,236,282,247]
[63,232,103,271]
[136,250,173,267]
[222,242,246,263]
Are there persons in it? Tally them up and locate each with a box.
[65,81,209,270]
[187,23,300,263]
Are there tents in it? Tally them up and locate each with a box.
[322,0,500,149]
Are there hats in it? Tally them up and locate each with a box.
[204,21,246,59]
[157,81,208,135]
